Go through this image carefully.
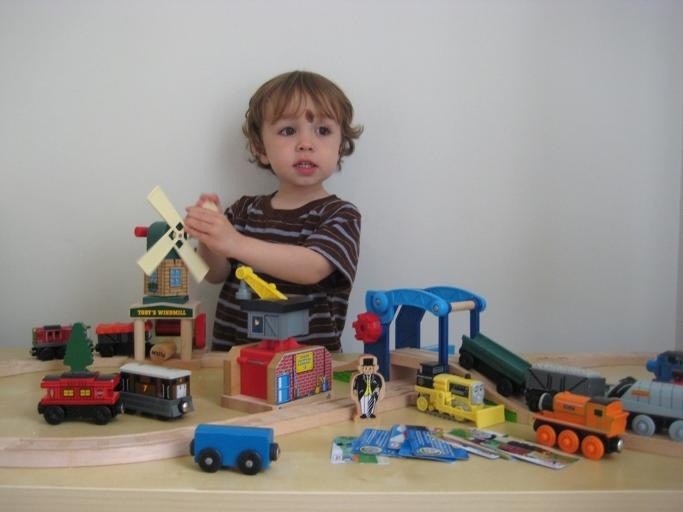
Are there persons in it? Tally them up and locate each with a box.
[183,70,366,352]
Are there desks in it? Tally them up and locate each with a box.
[0,345,683,512]
